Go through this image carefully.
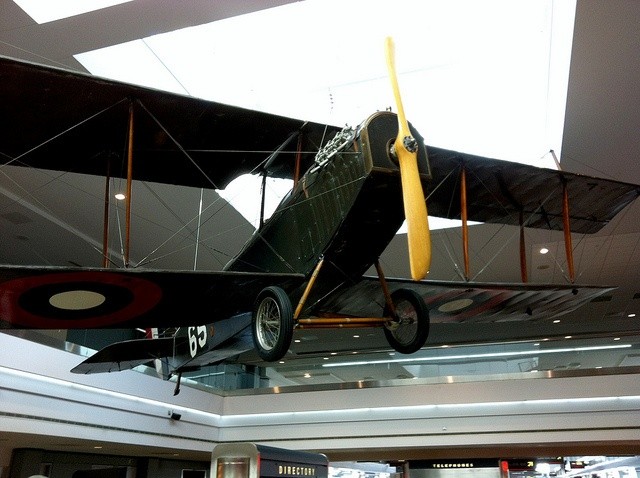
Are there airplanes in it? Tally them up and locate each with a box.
[0,36,640,396]
